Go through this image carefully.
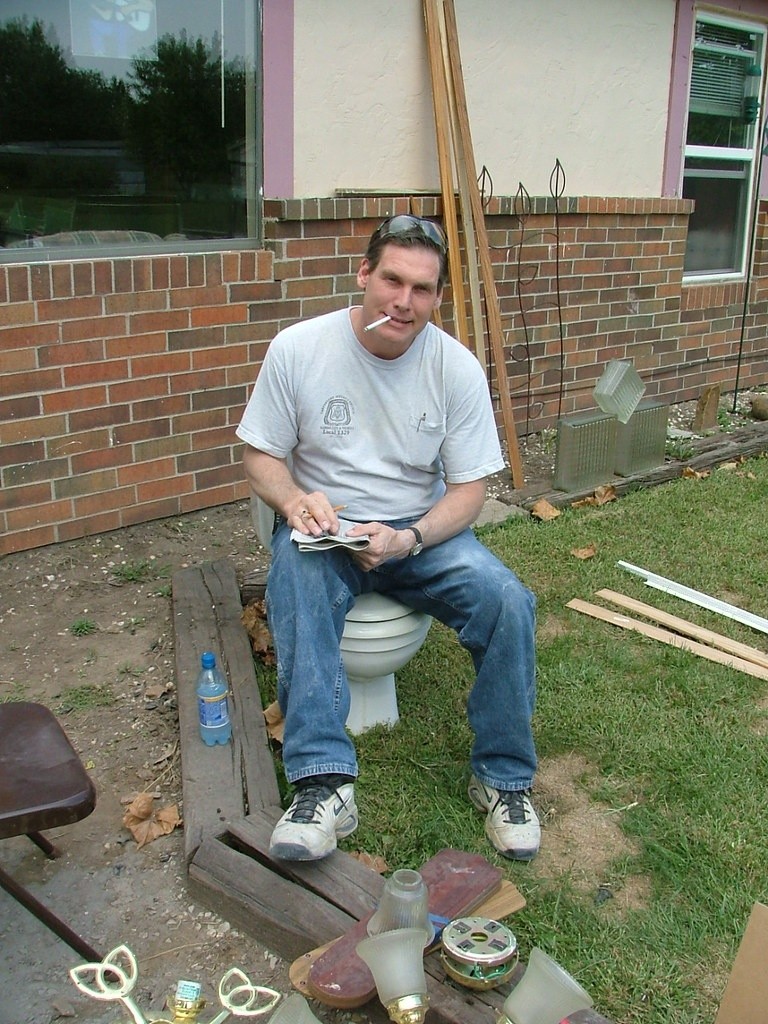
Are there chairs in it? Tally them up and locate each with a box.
[0,702,120,982]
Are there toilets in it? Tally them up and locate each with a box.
[250,491,435,739]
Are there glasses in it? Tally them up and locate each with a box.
[376,214,449,255]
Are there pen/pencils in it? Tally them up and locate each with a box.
[303,505,349,519]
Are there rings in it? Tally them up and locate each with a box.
[300,510,310,516]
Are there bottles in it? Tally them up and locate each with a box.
[194,651,233,747]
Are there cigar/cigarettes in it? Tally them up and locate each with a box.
[363,316,392,333]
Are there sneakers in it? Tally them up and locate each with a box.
[467,773,541,861]
[270,771,359,862]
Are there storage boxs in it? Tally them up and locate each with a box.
[553,358,668,493]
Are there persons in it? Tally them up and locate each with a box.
[236,216,542,860]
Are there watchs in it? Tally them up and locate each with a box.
[406,526,424,558]
